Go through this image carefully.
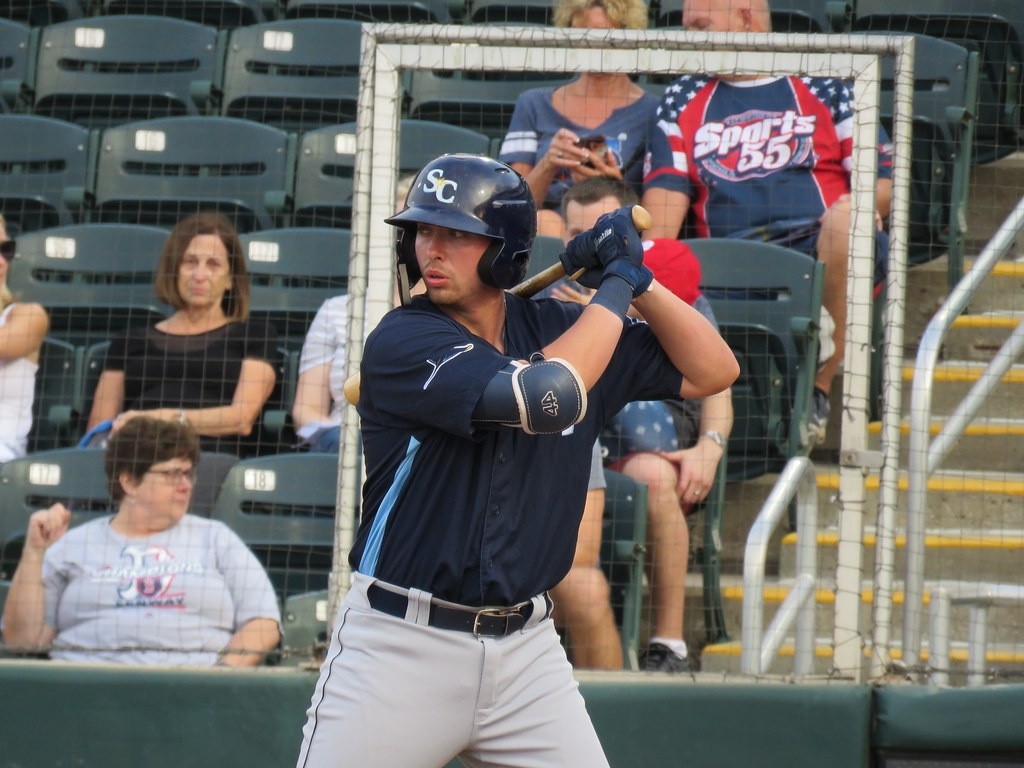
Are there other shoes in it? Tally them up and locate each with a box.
[808,389,832,445]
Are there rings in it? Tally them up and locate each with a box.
[558,152,562,157]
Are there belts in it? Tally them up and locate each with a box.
[368,583,553,639]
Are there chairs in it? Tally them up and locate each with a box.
[0,0,1024,768]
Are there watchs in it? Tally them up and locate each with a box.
[698,426,729,449]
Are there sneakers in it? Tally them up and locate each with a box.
[640,643,693,674]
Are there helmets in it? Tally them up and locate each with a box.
[382,153,538,307]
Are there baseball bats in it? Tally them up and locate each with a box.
[343,204,652,407]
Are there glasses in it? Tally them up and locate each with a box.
[0,240,16,260]
[146,468,197,488]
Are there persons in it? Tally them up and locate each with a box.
[0,417,284,668]
[84,209,284,459]
[297,151,741,768]
[498,0,661,246]
[641,1,893,446]
[0,215,50,470]
[290,174,734,677]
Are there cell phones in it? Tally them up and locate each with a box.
[574,134,608,170]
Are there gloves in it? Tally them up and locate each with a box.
[558,204,654,299]
